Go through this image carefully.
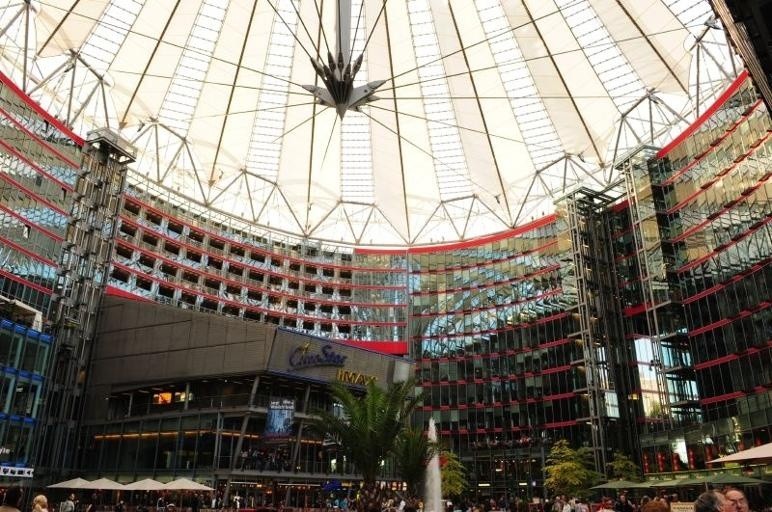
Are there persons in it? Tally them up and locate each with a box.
[1,487,772,512]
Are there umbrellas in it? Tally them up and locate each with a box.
[704,443,772,466]
[47,476,89,490]
[85,478,123,490]
[159,478,215,492]
[122,479,172,492]
